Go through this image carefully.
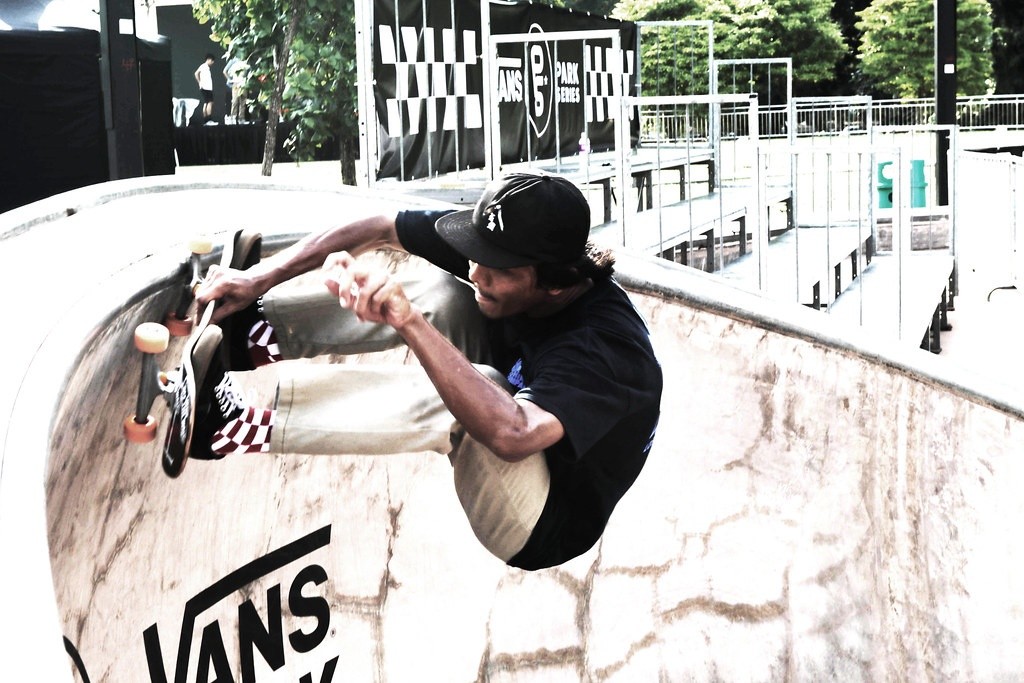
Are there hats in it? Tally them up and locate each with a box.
[433,172,591,267]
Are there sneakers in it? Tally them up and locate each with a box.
[213,228,265,372]
[187,324,243,461]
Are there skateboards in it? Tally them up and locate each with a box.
[125,229,246,481]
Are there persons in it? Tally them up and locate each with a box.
[580,132,590,153]
[185,172,664,572]
[194,55,219,126]
[224,58,250,125]
